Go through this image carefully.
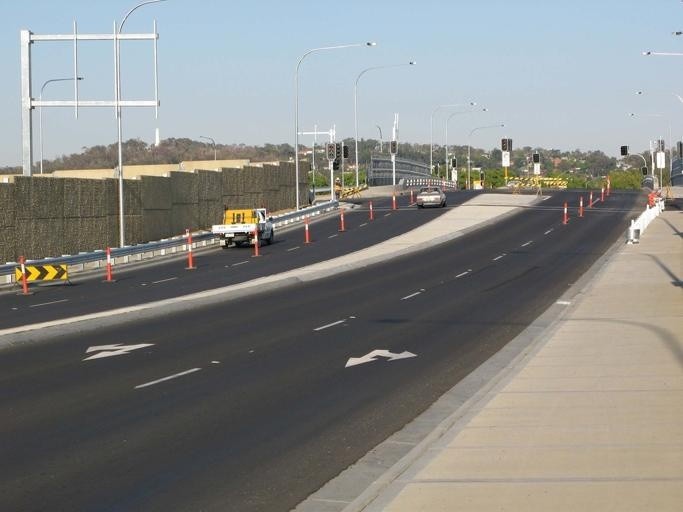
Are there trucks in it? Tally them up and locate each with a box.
[212,208,274,249]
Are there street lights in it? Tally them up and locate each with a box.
[628,51,683,188]
[621,146,627,155]
[39,78,84,174]
[293,39,505,213]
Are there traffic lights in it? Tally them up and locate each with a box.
[502,139,512,151]
